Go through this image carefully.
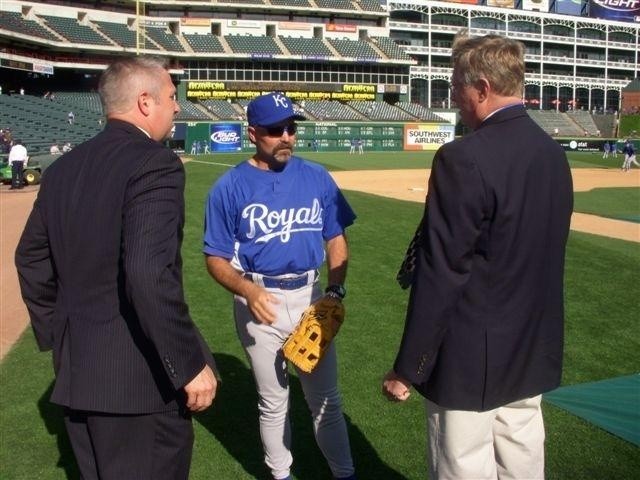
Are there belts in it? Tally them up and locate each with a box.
[243,268,319,290]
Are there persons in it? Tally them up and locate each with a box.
[190,139,211,155]
[311,136,319,153]
[349,138,364,155]
[381,28,574,479]
[50,141,73,155]
[15,57,222,480]
[1,127,29,189]
[67,111,74,126]
[204,94,358,480]
[601,139,640,171]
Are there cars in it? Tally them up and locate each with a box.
[0,151,61,184]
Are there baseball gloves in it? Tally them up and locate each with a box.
[283,297,345,375]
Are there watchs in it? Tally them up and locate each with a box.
[326,284,347,298]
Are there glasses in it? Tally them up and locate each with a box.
[268,123,298,136]
[446,81,459,93]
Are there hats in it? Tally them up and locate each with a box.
[247,93,307,127]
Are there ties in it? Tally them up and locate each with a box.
[395,216,422,289]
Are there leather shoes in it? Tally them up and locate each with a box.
[9,185,23,190]
